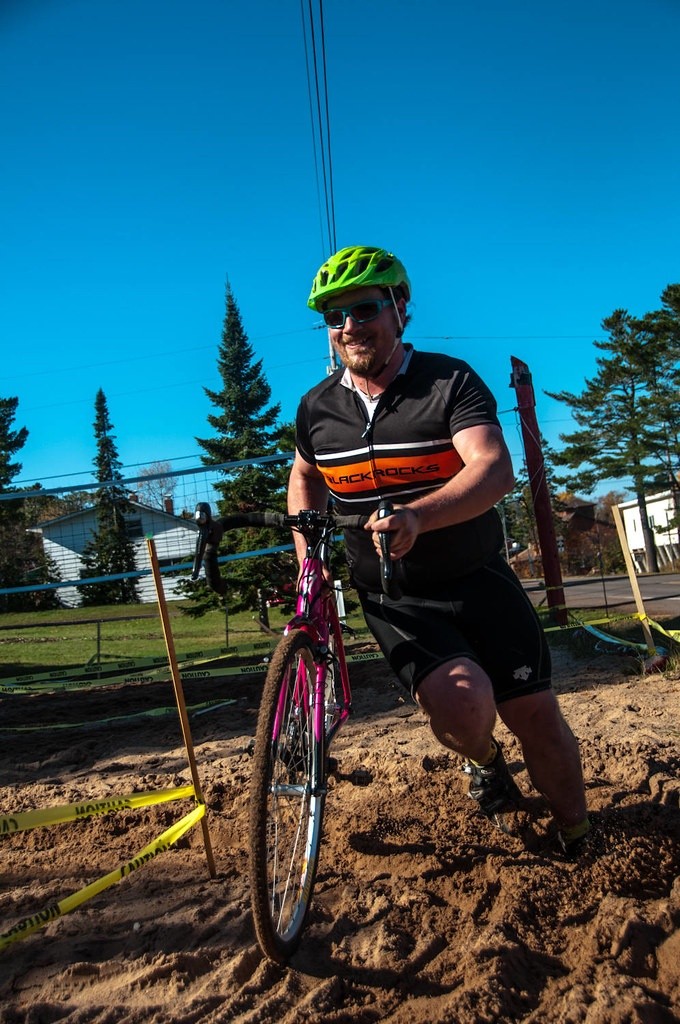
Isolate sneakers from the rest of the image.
[462,734,529,833]
[549,825,597,865]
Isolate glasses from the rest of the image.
[323,298,398,329]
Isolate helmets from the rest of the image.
[307,246,411,316]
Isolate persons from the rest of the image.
[286,246,592,855]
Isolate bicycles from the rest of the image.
[186,501,399,962]
